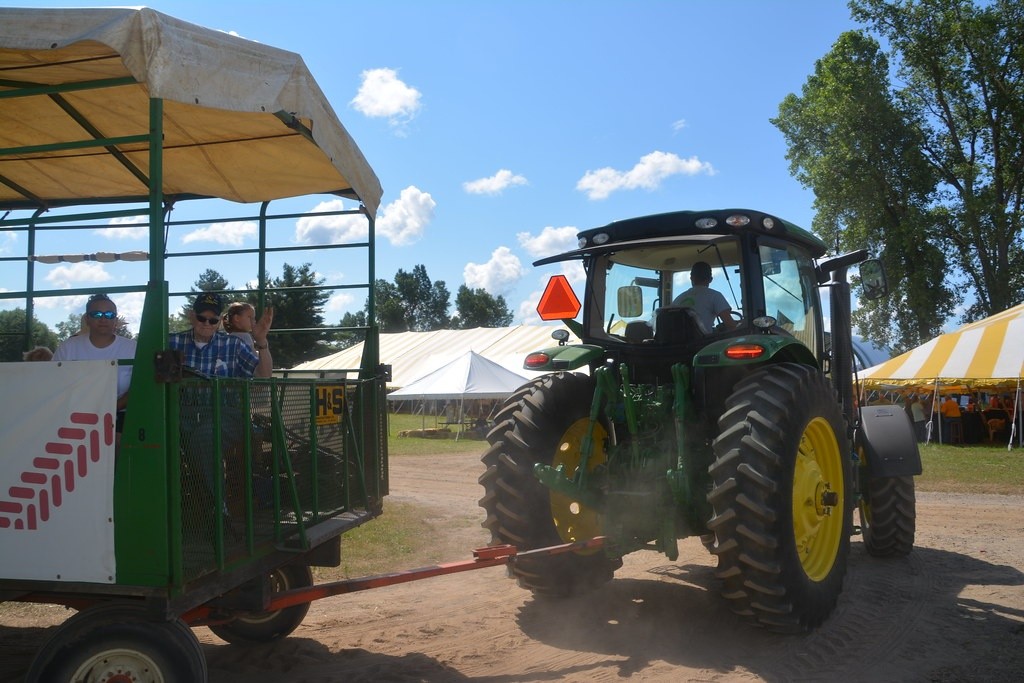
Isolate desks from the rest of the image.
[960,412,1022,444]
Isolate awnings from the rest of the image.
[289,319,628,439]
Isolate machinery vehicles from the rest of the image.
[0,7,924,683]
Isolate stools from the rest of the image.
[951,422,964,444]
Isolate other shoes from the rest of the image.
[210,499,231,534]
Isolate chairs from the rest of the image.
[652,307,709,341]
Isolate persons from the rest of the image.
[668,260,737,335]
[23,293,137,450]
[168,291,276,525]
[869,389,1024,447]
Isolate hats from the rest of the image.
[192,293,222,316]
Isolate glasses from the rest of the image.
[86,310,116,319]
[195,314,220,325]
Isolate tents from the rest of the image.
[852,303,1024,447]
